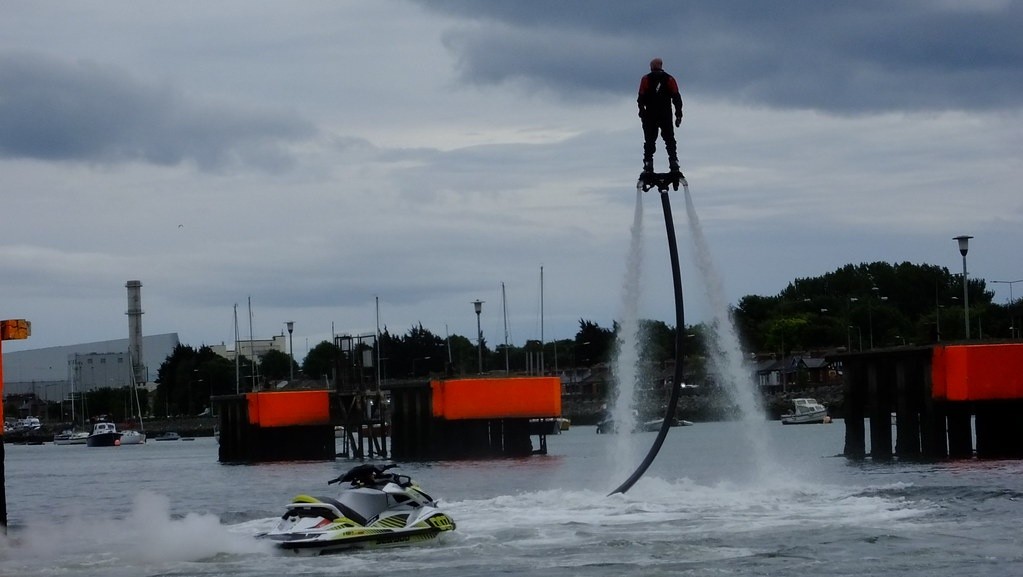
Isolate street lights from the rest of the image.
[673,333,696,380]
[990,279,1023,342]
[953,235,975,341]
[282,321,296,385]
[568,340,591,400]
[935,271,970,342]
[781,298,812,396]
[847,296,859,354]
[412,356,432,380]
[869,286,880,350]
[469,300,487,378]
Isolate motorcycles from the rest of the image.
[594,412,639,435]
[253,462,458,559]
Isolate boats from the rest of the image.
[643,414,693,433]
[780,398,833,426]
[154,431,181,441]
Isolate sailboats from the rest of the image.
[213,295,264,445]
[526,340,572,431]
[5,345,146,447]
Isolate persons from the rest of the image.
[637,57,683,173]
